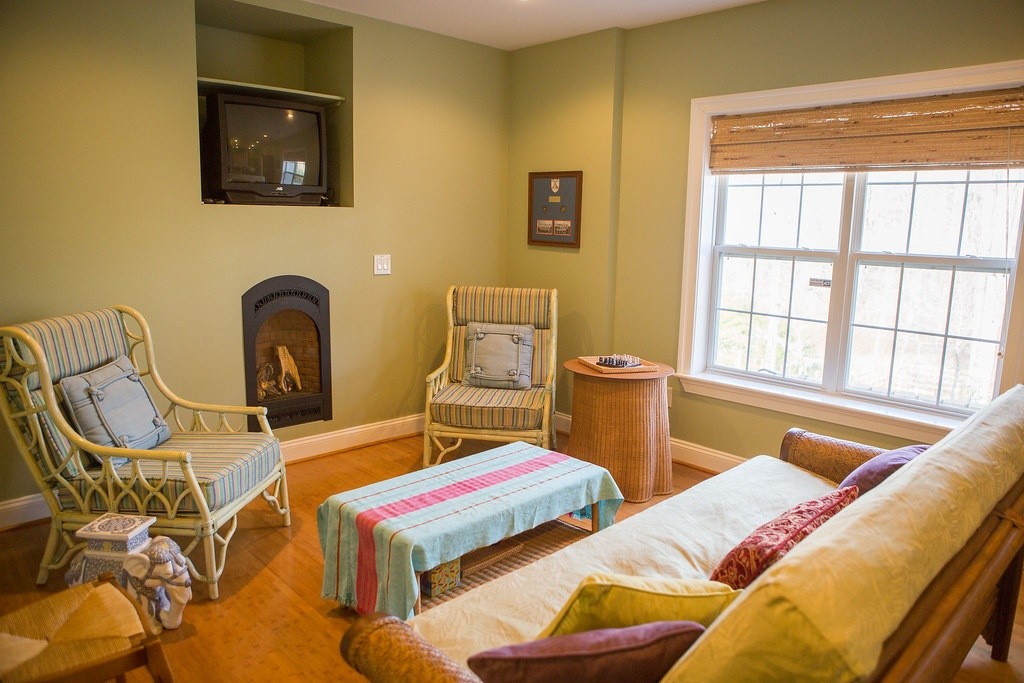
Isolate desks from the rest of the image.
[563,359,675,503]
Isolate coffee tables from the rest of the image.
[317,441,625,622]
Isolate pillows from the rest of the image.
[59,355,171,469]
[467,621,706,683]
[535,574,743,629]
[461,322,535,391]
[710,485,860,591]
[837,445,931,497]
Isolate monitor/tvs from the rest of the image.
[198,92,329,207]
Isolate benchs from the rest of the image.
[339,382,1023,683]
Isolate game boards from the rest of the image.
[578,353,659,373]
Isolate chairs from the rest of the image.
[0,306,291,600]
[422,286,558,467]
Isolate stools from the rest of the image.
[1,571,175,683]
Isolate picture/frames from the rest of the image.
[528,171,583,247]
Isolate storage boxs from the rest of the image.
[420,557,461,597]
